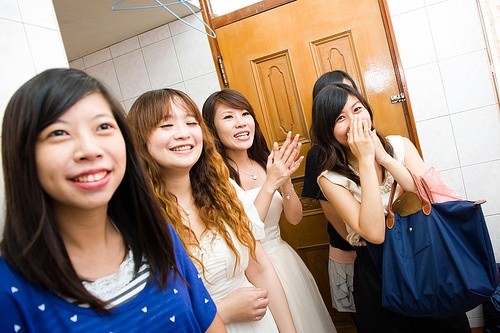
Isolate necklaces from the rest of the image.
[236,158,258,180]
[177,192,193,215]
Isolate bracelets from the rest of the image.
[280,184,296,202]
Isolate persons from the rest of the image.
[0,68,227,333]
[316,81,468,333]
[125,88,297,333]
[301,70,359,333]
[202,89,339,333]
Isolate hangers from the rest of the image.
[154,0,217,39]
[110,0,188,10]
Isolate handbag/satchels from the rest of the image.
[383,167,499,317]
